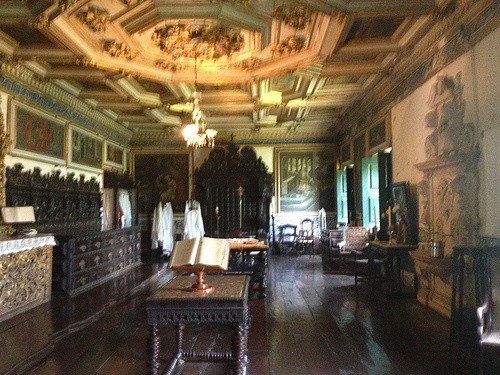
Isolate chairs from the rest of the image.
[473,245,500,375]
[278,217,315,255]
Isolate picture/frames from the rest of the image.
[104,139,127,170]
[66,121,105,174]
[363,114,391,157]
[274,146,338,216]
[129,149,193,218]
[339,139,353,164]
[6,94,68,166]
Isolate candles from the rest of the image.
[389,206,391,227]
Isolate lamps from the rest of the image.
[181,48,217,148]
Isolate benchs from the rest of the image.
[320,226,370,271]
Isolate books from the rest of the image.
[169,237,230,270]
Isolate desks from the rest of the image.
[224,233,270,299]
[370,241,419,298]
[146,273,253,375]
